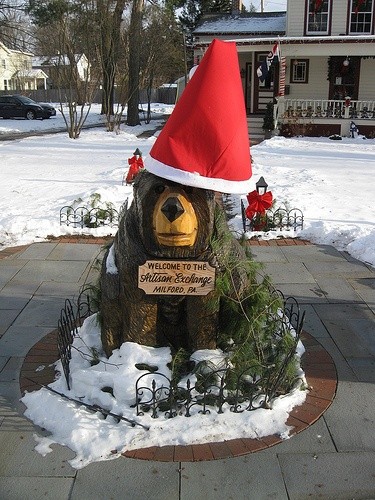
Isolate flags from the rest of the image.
[257,34,286,103]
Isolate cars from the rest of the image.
[21,95,56,119]
[0,95,44,120]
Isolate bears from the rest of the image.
[98,171,250,374]
